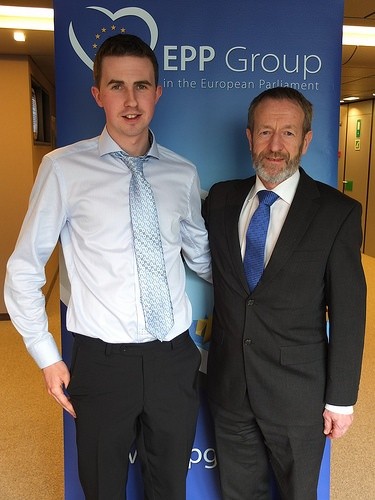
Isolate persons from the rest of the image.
[4,33,213,500]
[201,87,367,500]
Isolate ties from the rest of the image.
[243,190,279,293]
[110,150,175,342]
